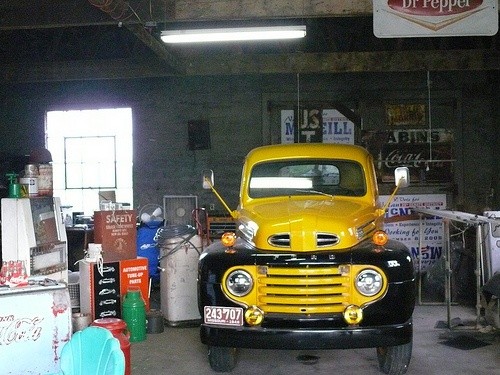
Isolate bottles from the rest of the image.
[19,162,40,196]
[0,259,27,282]
[37,161,54,196]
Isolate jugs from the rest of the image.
[145,308,165,335]
[89,318,131,375]
[121,289,147,342]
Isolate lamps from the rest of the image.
[160,0,307,44]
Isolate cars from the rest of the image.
[196,141,418,374]
